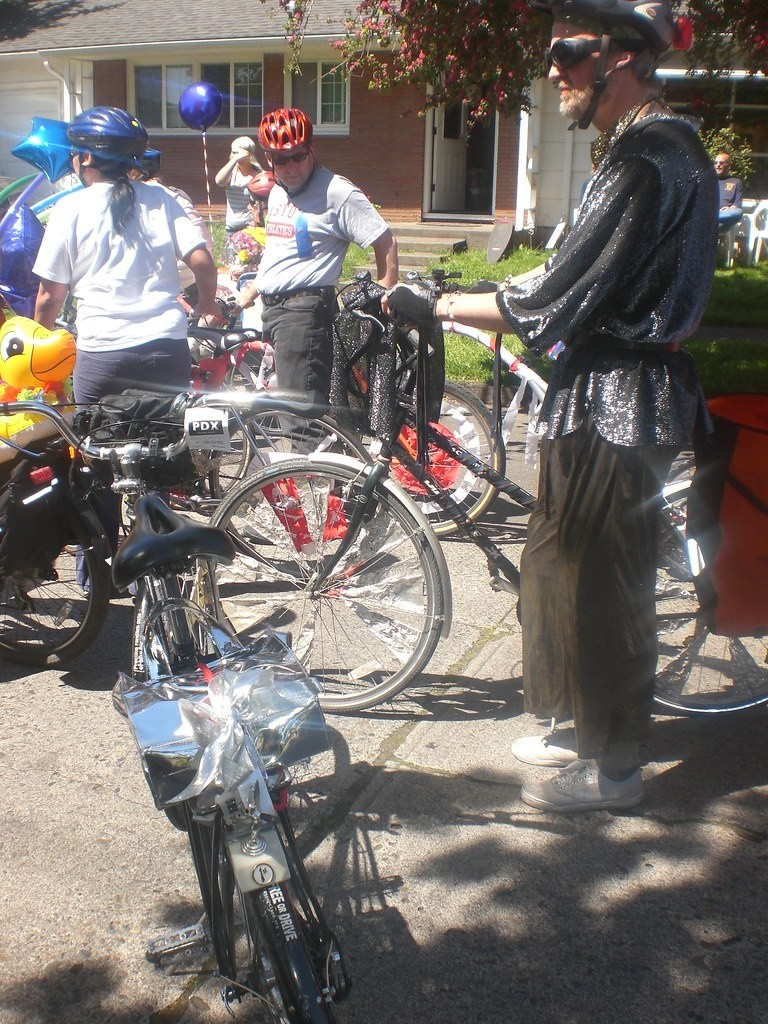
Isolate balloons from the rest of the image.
[12,116,79,182]
[0,201,45,320]
[179,81,221,133]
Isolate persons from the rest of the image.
[30,107,227,599]
[380,0,721,813]
[215,136,260,268]
[127,145,217,292]
[216,172,277,297]
[229,108,398,491]
[713,152,743,235]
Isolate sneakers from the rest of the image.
[521,758,645,814]
[511,717,581,766]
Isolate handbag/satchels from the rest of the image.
[111,596,331,811]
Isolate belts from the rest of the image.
[261,285,335,305]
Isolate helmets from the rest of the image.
[246,171,278,201]
[143,146,161,160]
[530,0,676,59]
[258,107,313,153]
[67,105,149,144]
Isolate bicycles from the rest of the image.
[0,397,354,1024]
[0,266,768,730]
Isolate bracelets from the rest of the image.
[447,289,463,321]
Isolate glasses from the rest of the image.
[544,37,593,72]
[273,150,310,165]
[249,199,255,206]
[70,143,78,156]
